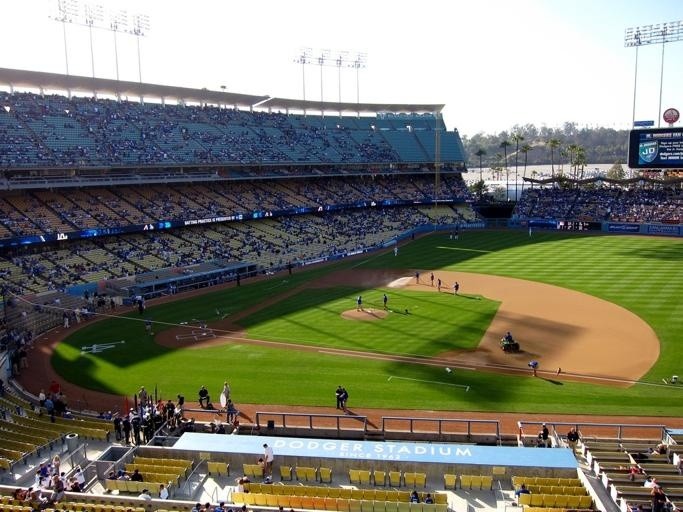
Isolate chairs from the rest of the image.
[356,112,481,255]
[193,105,355,289]
[0,346,35,511]
[0,88,193,342]
[36,395,683,511]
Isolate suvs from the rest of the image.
[499,337,519,352]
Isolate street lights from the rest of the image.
[46,0,151,84]
[623,17,682,132]
[291,45,369,116]
